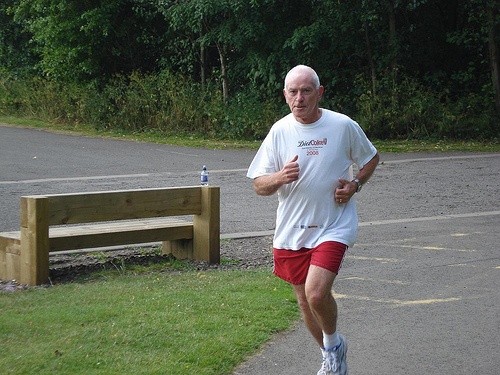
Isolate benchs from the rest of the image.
[0,185,220,286]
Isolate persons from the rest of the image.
[245,64,381,375]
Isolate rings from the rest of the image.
[339,198,343,203]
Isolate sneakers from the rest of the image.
[315,335,349,375]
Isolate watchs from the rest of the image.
[350,178,362,192]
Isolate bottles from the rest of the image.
[201,165,208,186]
